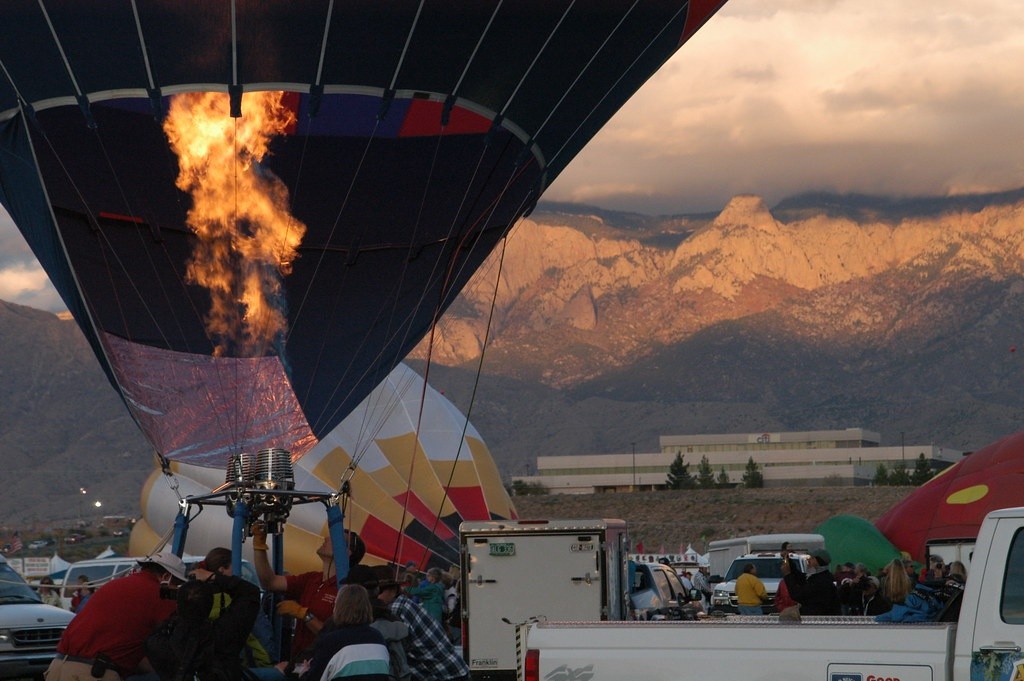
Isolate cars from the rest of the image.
[0,552,78,681]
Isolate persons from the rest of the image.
[34,531,471,681]
[678,541,968,623]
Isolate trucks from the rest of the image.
[708,532,825,580]
[457,517,628,674]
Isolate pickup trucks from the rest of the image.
[522,505,1024,681]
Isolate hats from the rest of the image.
[135,551,188,585]
[364,566,402,590]
[806,548,832,566]
[699,559,711,568]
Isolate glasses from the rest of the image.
[378,584,386,595]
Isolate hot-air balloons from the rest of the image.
[0,2,732,677]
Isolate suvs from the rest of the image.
[711,550,814,609]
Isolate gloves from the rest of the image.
[250,522,270,550]
[276,599,309,620]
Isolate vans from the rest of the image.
[57,555,260,613]
[627,560,703,622]
